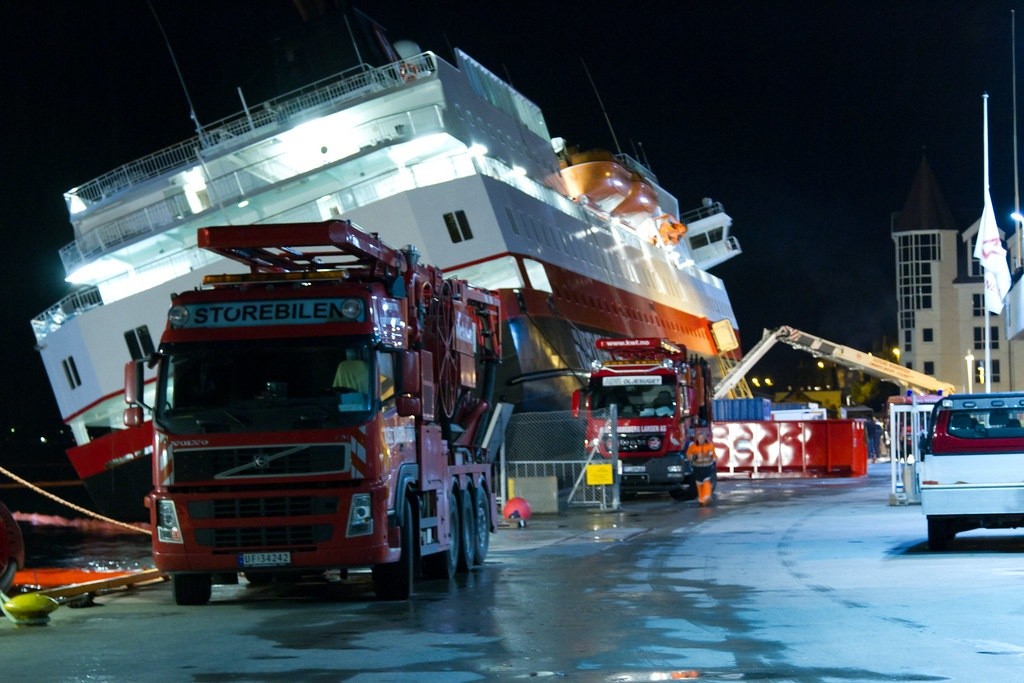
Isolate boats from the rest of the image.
[34,31,740,517]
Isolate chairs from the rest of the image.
[950,411,1024,439]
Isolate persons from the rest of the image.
[883,417,891,457]
[684,433,718,507]
[863,414,879,457]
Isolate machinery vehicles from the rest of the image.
[712,325,955,462]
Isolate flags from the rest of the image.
[971,122,1014,317]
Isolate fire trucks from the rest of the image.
[124,216,501,607]
[570,339,716,505]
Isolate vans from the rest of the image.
[919,394,1024,544]
